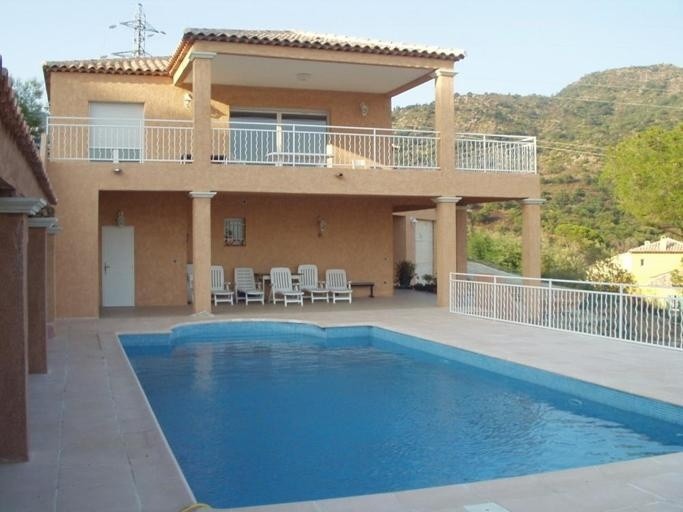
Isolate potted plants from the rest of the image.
[395,260,415,288]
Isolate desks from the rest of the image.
[254,272,303,305]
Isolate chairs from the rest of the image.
[211,265,234,307]
[326,269,353,303]
[234,268,265,306]
[270,267,304,307]
[298,264,329,304]
